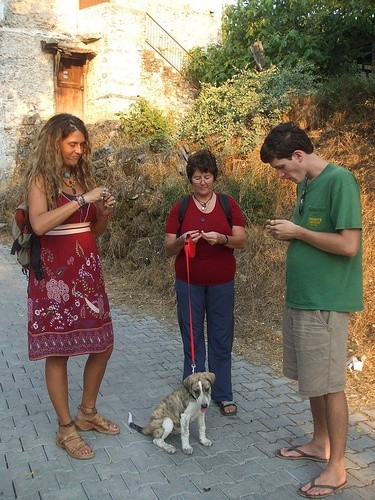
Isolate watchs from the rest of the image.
[222,235,228,245]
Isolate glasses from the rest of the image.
[299,186,306,216]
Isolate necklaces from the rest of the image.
[194,193,213,210]
[62,172,77,194]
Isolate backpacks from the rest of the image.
[11,171,58,270]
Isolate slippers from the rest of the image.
[215,401,237,416]
[275,445,329,462]
[296,475,348,499]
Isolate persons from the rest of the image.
[26,113,121,459]
[261,122,363,498]
[165,150,248,417]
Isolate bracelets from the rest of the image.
[74,195,85,207]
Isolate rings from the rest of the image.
[102,197,104,200]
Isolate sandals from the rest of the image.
[75,406,120,434]
[56,422,94,459]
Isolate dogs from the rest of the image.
[127,372,216,455]
